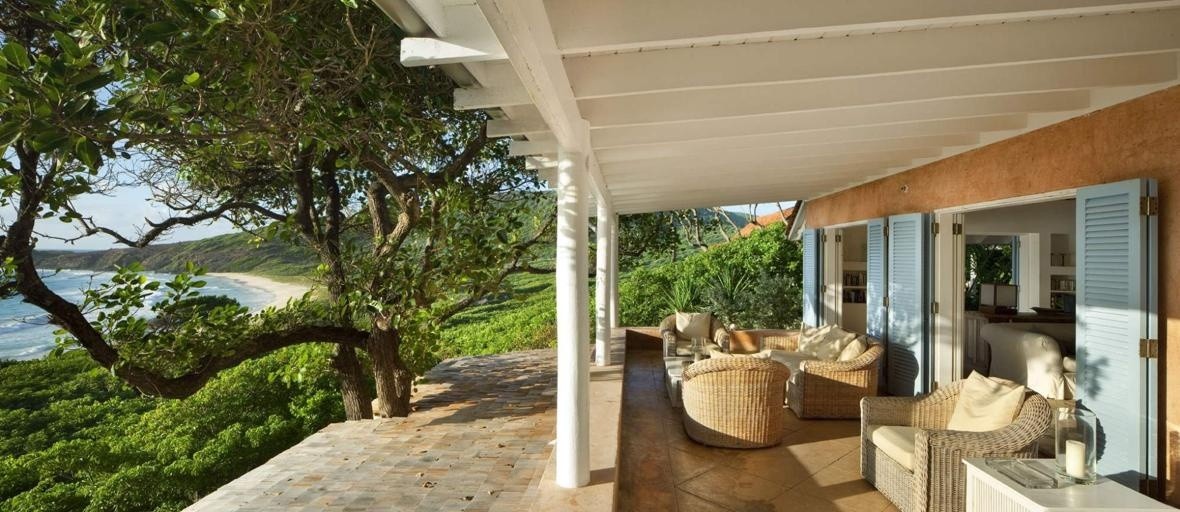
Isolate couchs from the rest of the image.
[759,326,886,421]
[980,322,1076,401]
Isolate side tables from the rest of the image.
[960,458,1180,512]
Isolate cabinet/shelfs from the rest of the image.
[1050,266,1076,311]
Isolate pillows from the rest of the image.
[947,369,1025,432]
[1062,356,1075,372]
[794,322,868,362]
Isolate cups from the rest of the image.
[690,336,707,363]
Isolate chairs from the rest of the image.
[859,376,1052,512]
[659,313,791,450]
